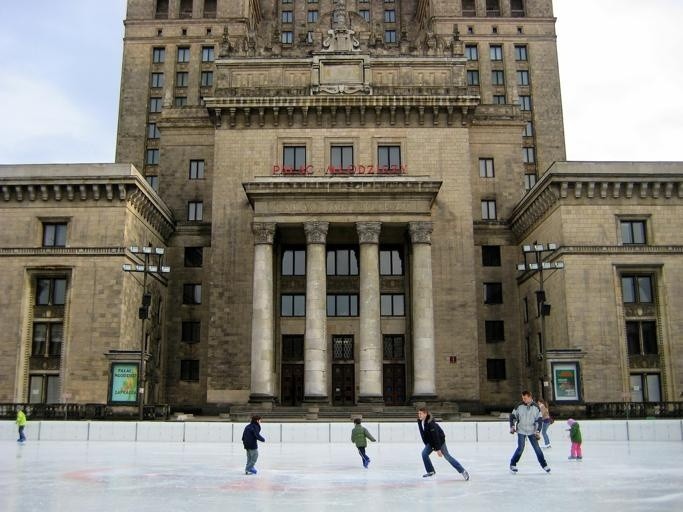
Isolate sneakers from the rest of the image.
[18,437,25,442]
[461,470,468,478]
[245,467,256,473]
[422,470,434,477]
[364,460,369,467]
[510,444,582,473]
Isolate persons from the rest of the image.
[537,399,551,448]
[510,391,550,472]
[417,408,469,480]
[15,405,26,442]
[566,418,582,459]
[351,418,376,468]
[242,415,265,474]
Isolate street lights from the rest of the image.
[516,240,565,411]
[121,243,172,420]
